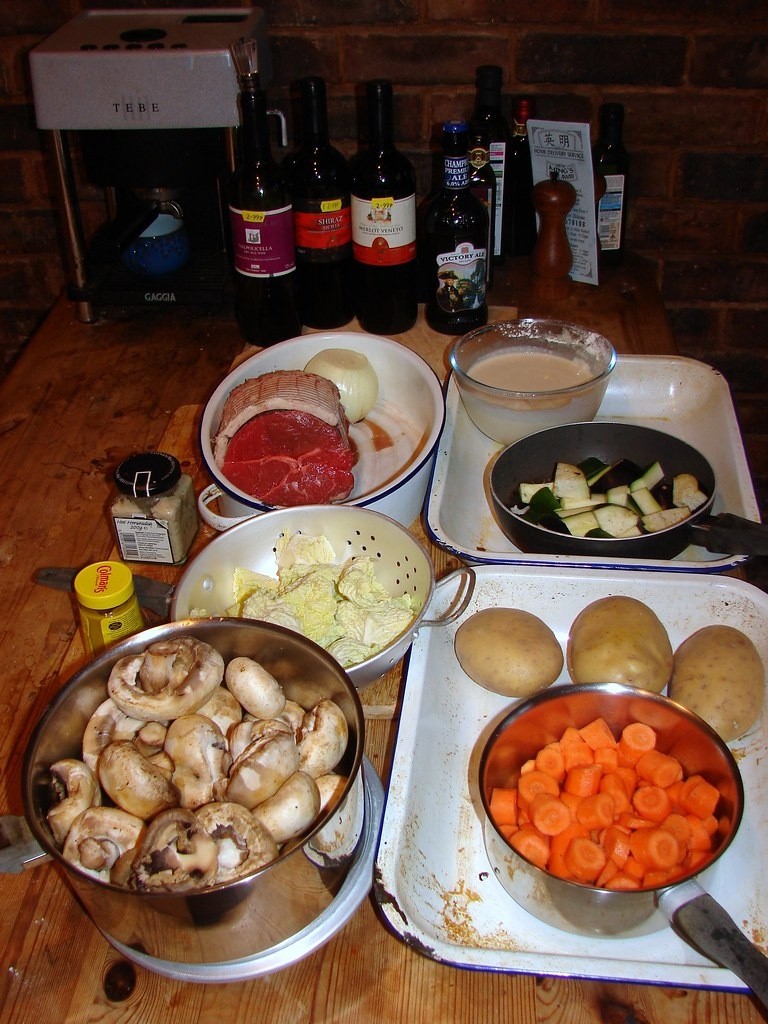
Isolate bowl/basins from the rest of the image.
[448,318,617,445]
[197,331,446,529]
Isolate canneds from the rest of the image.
[75,562,144,656]
[108,451,200,565]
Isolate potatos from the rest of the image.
[456,595,764,742]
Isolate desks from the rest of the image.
[0,256,768,1024]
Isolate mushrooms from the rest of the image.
[49,636,348,892]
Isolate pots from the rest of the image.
[33,505,476,692]
[1,617,364,902]
[478,681,767,1017]
[488,422,768,559]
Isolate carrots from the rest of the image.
[487,716,720,889]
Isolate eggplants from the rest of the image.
[515,456,707,539]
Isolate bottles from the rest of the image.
[292,77,354,329]
[416,124,443,303]
[465,129,496,290]
[350,78,417,334]
[508,99,536,257]
[529,171,577,279]
[111,451,201,565]
[588,104,629,268]
[123,186,192,281]
[426,122,489,335]
[227,37,296,293]
[72,561,146,659]
[469,66,510,264]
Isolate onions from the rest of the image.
[304,349,378,423]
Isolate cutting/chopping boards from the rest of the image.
[54,403,433,721]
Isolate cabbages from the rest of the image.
[226,529,413,667]
